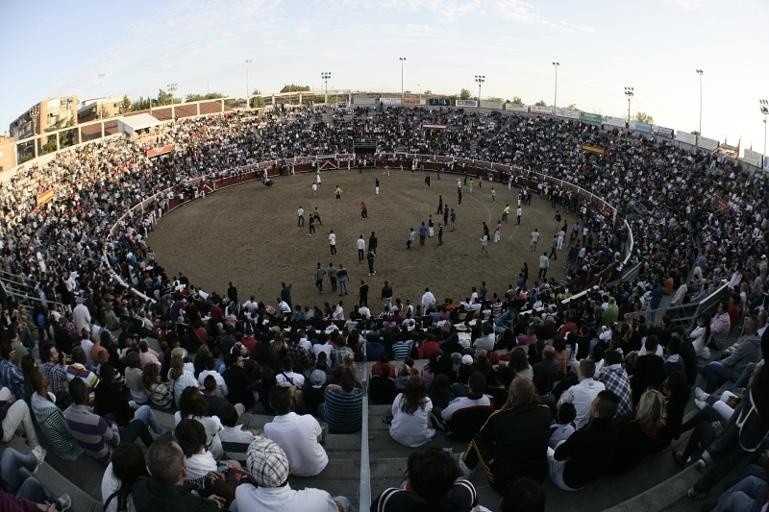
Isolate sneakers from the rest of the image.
[31,445,46,473]
[694,387,710,400]
[693,399,707,409]
[51,492,72,511]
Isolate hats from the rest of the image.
[462,353,474,365]
[170,347,189,361]
[310,368,327,389]
[247,436,289,488]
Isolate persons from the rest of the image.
[1,102,769,511]
